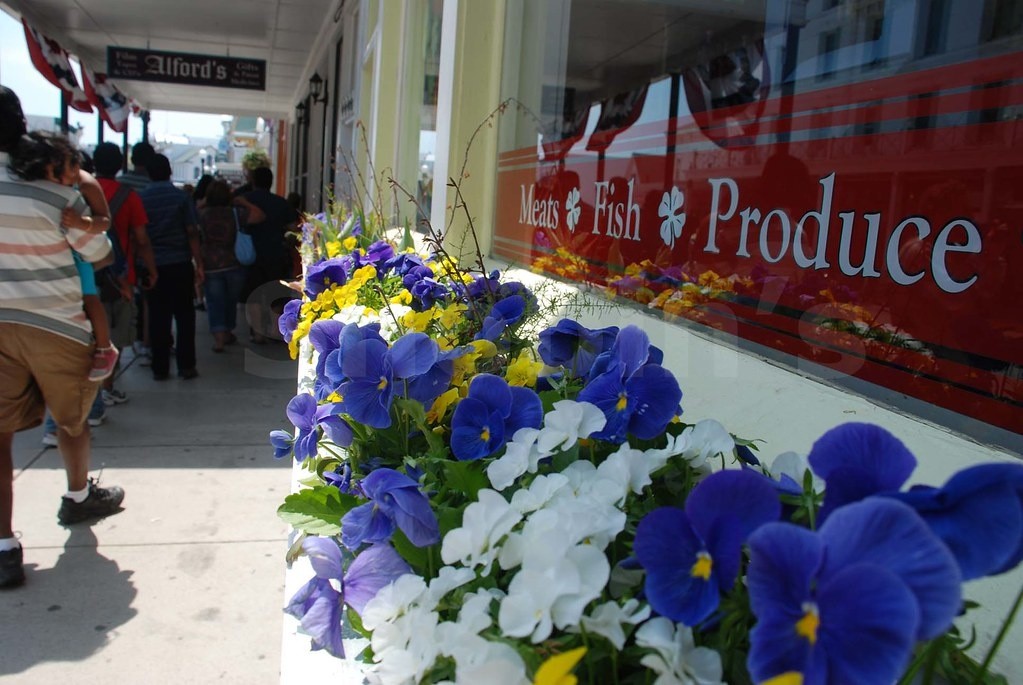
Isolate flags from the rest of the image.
[19,17,94,113]
[78,55,148,133]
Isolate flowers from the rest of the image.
[279,96,1023,685]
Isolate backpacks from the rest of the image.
[94,184,133,304]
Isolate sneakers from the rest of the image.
[58,484,124,523]
[43,433,57,446]
[103,389,130,405]
[1,542,24,585]
[88,411,106,426]
[88,342,119,382]
[138,344,154,366]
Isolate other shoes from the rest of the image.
[152,371,198,380]
[194,299,204,309]
[250,335,264,343]
[212,335,238,352]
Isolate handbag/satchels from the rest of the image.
[233,208,257,267]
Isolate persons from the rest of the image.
[117,138,162,367]
[231,163,299,345]
[84,139,157,408]
[176,164,304,310]
[41,385,110,450]
[74,147,96,176]
[2,126,120,383]
[193,173,265,355]
[0,82,125,588]
[531,136,1022,439]
[135,152,206,386]
